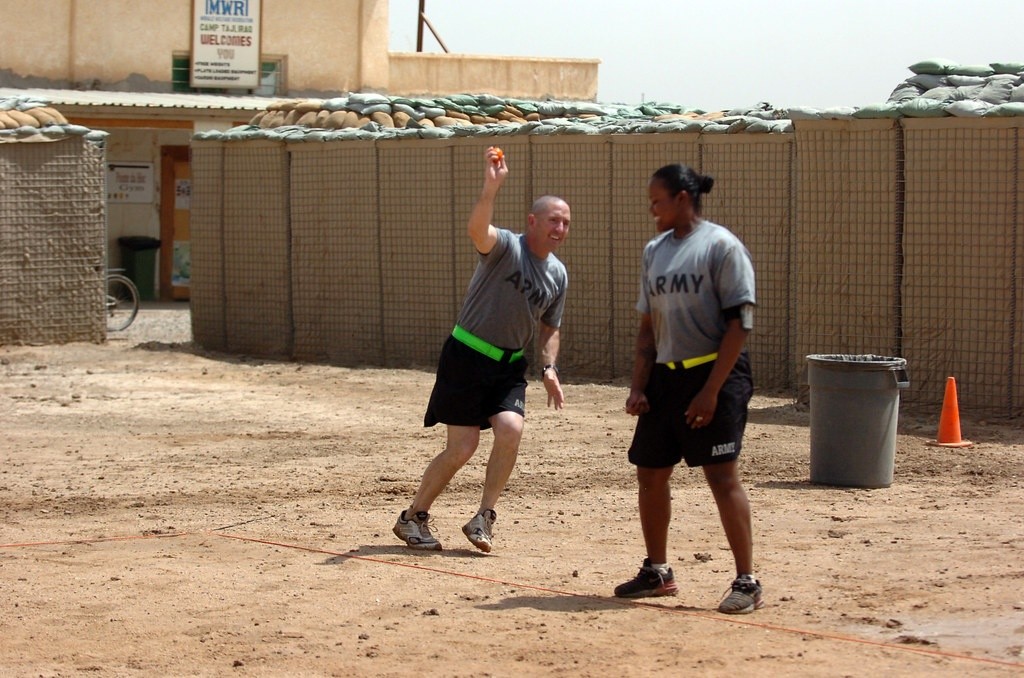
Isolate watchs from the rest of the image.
[542,364,559,377]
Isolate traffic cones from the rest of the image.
[927,377,973,448]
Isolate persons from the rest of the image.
[392,146,570,553]
[614,164,766,615]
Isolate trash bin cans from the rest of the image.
[117,235,161,301]
[805,354,910,489]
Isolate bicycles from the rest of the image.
[105,267,140,333]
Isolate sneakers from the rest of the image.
[393,510,443,550]
[614,557,678,598]
[463,509,497,552]
[719,579,764,613]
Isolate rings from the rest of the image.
[696,416,703,421]
